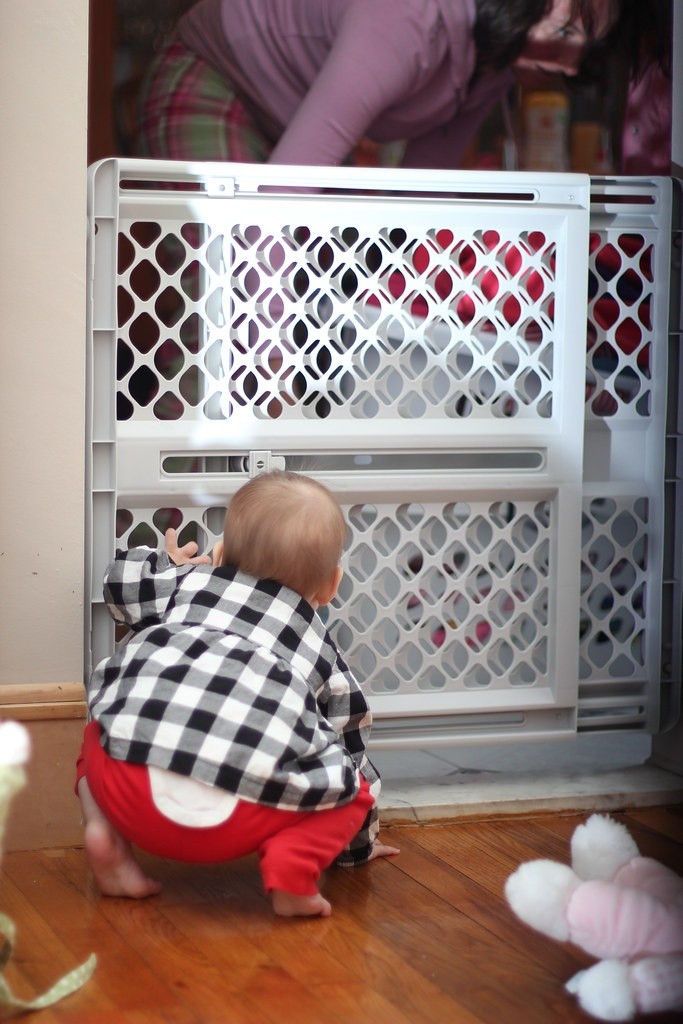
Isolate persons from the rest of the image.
[115,1,648,565]
[65,472,402,919]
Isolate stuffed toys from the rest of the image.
[499,814,682,1023]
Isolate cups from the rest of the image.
[524,90,568,172]
